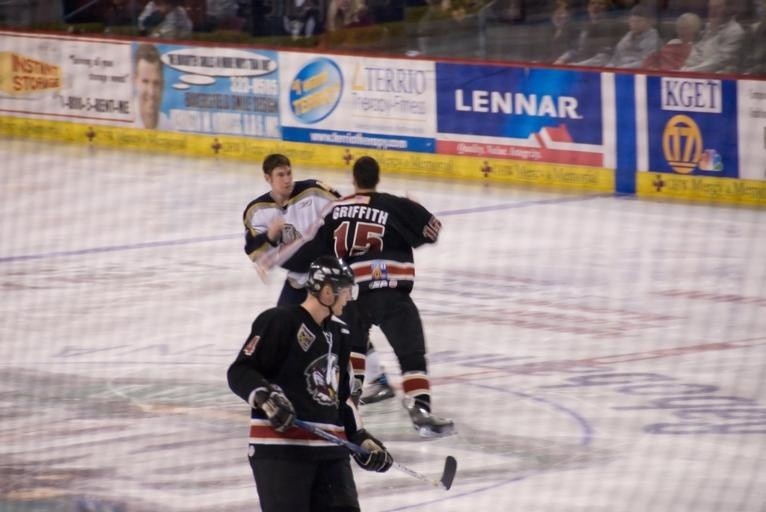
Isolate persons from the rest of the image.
[223,252,393,512]
[131,42,178,134]
[1,2,765,81]
[251,156,454,436]
[240,152,393,401]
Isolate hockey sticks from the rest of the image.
[292,420,457,490]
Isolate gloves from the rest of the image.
[349,428,394,474]
[253,383,295,433]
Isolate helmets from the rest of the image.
[306,255,355,295]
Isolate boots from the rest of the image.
[401,395,454,435]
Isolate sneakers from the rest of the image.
[356,374,396,404]
